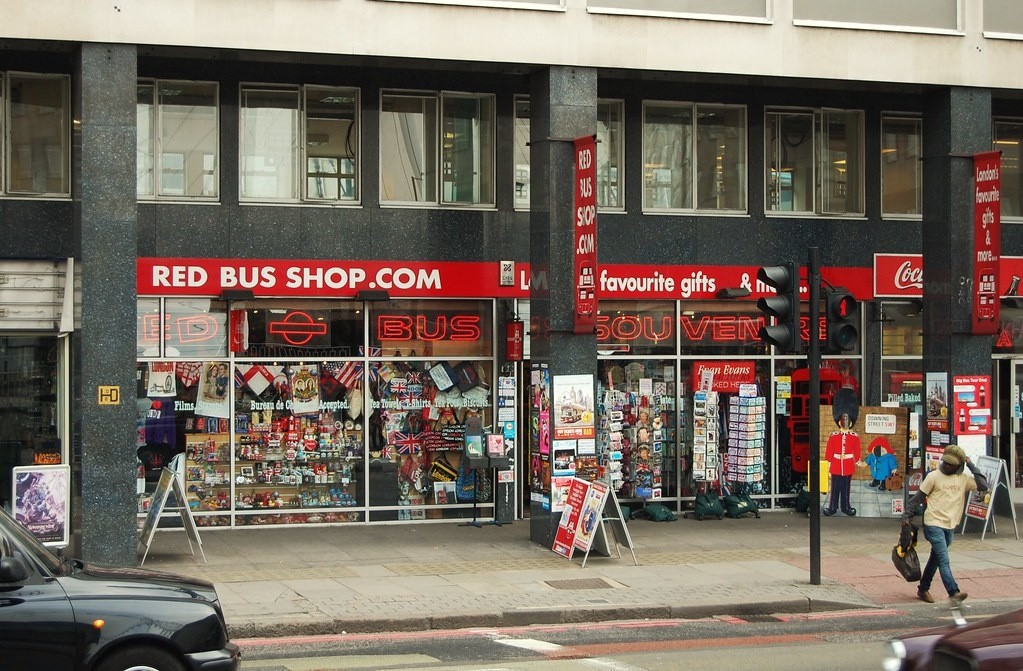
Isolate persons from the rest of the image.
[901,444,989,610]
[215,363,228,398]
[295,380,304,393]
[637,427,650,445]
[205,365,218,400]
[637,444,650,463]
[305,378,314,393]
[636,410,650,426]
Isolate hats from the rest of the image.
[940,444,967,465]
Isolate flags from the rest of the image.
[235,372,245,390]
[354,362,379,383]
[325,361,361,391]
[380,370,424,463]
[235,364,284,396]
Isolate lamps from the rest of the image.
[819,286,849,299]
[715,287,750,299]
[353,290,393,302]
[210,288,255,302]
[1001,297,1023,310]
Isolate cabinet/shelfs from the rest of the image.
[185,432,362,524]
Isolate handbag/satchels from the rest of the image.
[457,455,479,502]
[402,456,422,485]
[452,361,479,392]
[377,364,423,406]
[475,470,492,503]
[428,453,457,482]
[395,426,422,453]
[442,411,467,441]
[424,409,464,452]
[891,518,921,582]
[429,361,459,392]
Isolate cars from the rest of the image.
[881,607,1023,671]
[0,502,240,671]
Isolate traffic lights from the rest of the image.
[755,260,802,354]
[825,289,859,352]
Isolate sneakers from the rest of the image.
[948,592,968,601]
[917,585,935,603]
